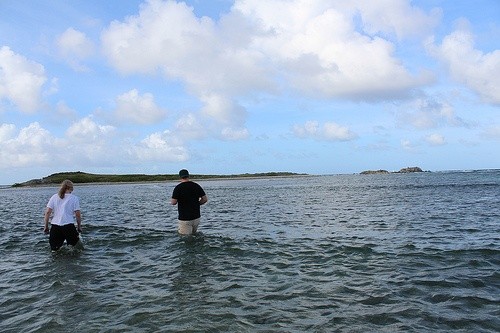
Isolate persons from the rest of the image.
[43,178,83,256]
[170,169,207,236]
